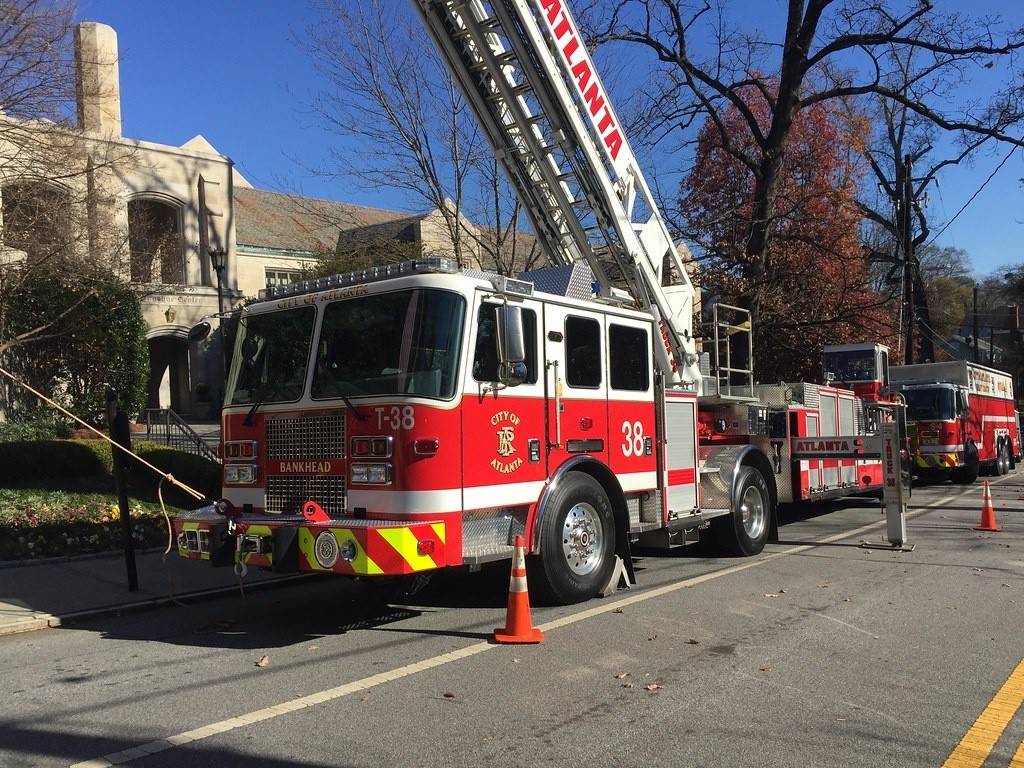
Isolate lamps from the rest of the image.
[165,306,177,321]
[823,372,834,386]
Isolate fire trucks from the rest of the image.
[820,341,1019,486]
[171,0,920,608]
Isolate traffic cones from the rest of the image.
[973,480,1004,531]
[494,533,544,644]
